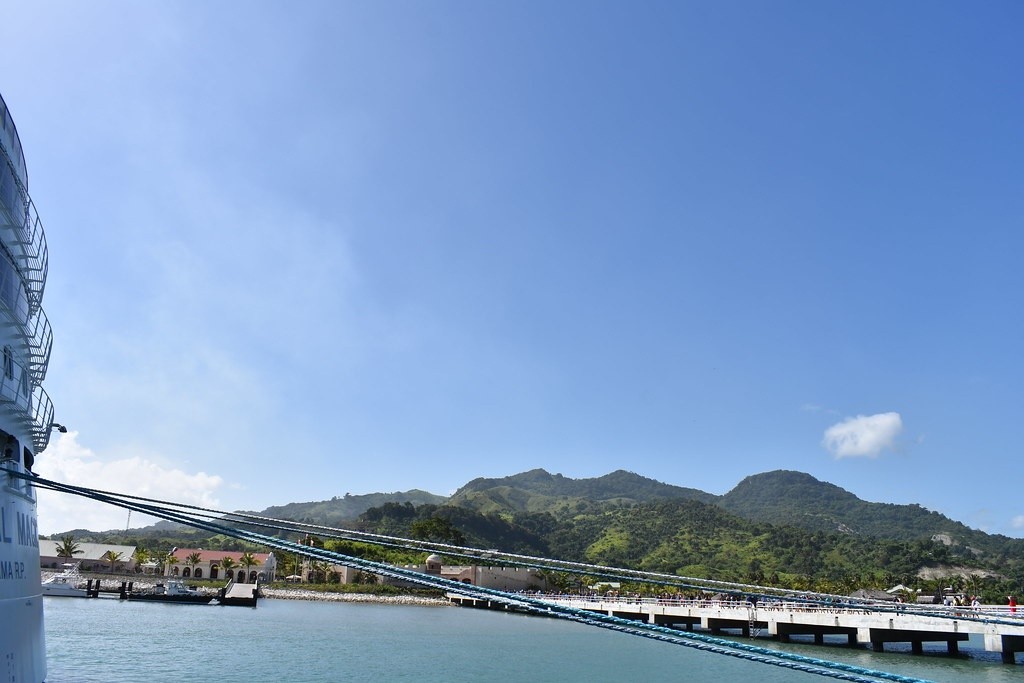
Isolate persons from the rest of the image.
[1008,596,1016,612]
[508,589,906,615]
[971,597,980,618]
[943,595,969,617]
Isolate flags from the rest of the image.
[298,533,314,546]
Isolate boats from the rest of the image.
[125,570,220,603]
[41,558,94,598]
[0,87,66,682]
